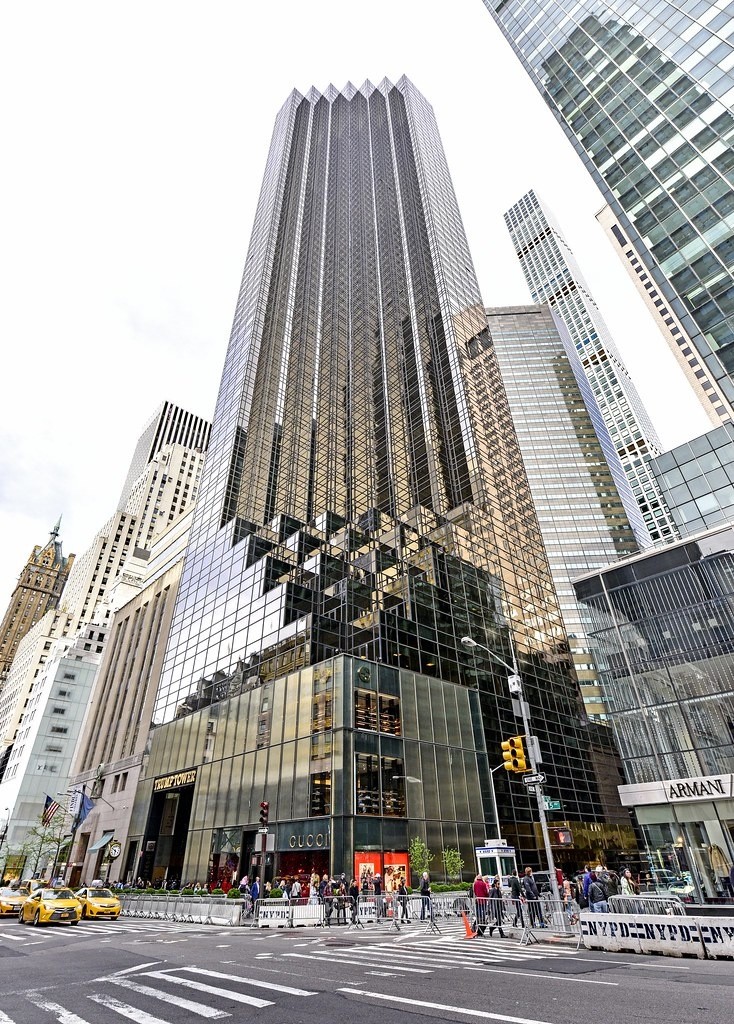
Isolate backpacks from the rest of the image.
[239,884,246,893]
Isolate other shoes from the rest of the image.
[350,918,355,924]
[611,932,615,936]
[602,932,607,936]
[489,927,493,937]
[407,920,411,924]
[501,934,508,938]
[401,920,405,923]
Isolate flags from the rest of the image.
[42,795,60,826]
[71,793,95,833]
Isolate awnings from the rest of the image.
[87,832,114,853]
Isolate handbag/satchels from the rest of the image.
[297,892,302,897]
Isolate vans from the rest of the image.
[19,879,50,894]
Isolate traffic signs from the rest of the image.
[522,772,561,811]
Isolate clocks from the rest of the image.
[104,840,121,861]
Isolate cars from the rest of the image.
[74,887,122,921]
[18,886,82,925]
[0,887,31,918]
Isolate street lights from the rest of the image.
[61,789,84,887]
[0,808,11,849]
[50,792,71,881]
[461,637,574,937]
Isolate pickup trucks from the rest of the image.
[635,869,678,892]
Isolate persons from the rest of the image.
[80,877,176,892]
[182,876,260,915]
[708,843,734,897]
[558,865,643,936]
[471,867,548,938]
[397,879,411,924]
[371,873,383,924]
[264,872,368,926]
[361,865,405,895]
[420,872,438,923]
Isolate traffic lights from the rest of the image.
[554,829,574,845]
[260,801,268,823]
[508,737,526,770]
[501,740,513,770]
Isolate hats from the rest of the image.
[113,881,118,883]
[589,873,597,881]
